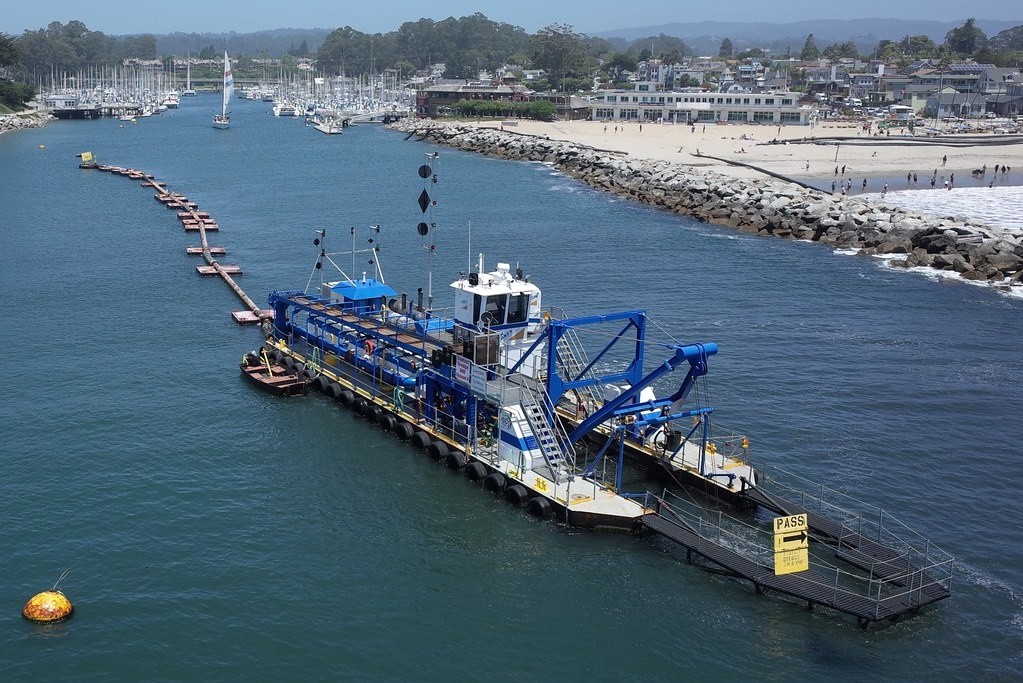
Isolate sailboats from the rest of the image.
[238,62,407,134]
[212,50,235,130]
[41,60,195,118]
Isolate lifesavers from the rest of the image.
[280,356,294,369]
[410,431,432,448]
[503,484,529,506]
[443,451,467,470]
[351,397,368,412]
[268,350,284,362]
[427,441,449,461]
[483,473,508,493]
[338,390,355,407]
[364,340,373,354]
[365,405,383,422]
[292,362,304,373]
[326,383,343,400]
[464,461,488,482]
[524,496,553,518]
[313,376,329,393]
[258,345,273,360]
[396,421,414,441]
[377,413,398,432]
[301,370,315,387]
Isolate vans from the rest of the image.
[849,98,863,107]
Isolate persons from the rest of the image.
[973,164,1010,174]
[841,185,846,195]
[842,165,846,175]
[848,178,852,190]
[913,173,918,183]
[703,125,705,132]
[615,126,617,133]
[862,178,867,190]
[832,181,835,193]
[908,173,911,182]
[640,125,642,132]
[931,169,954,189]
[881,182,888,198]
[863,125,904,137]
[691,125,695,133]
[721,133,756,153]
[806,160,809,171]
[834,166,838,176]
[604,125,607,132]
[943,155,948,166]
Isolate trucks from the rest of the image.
[815,92,827,101]
[830,95,845,105]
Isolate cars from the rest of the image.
[916,118,925,127]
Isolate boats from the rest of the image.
[265,148,750,530]
[238,345,313,396]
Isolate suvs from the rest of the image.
[981,112,997,119]
[942,116,966,124]
